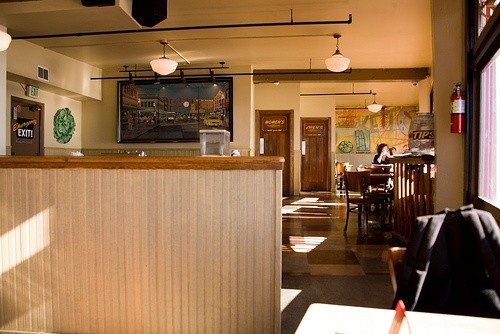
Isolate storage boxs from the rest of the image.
[199,129,231,158]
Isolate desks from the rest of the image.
[295,303,500,334]
[370,173,394,177]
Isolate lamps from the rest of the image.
[181,71,185,81]
[367,93,382,112]
[130,73,134,86]
[0,30,11,52]
[150,39,178,76]
[154,72,160,85]
[325,34,351,72]
[211,70,217,87]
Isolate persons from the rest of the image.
[373,144,396,198]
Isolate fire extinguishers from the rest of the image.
[450,84,466,134]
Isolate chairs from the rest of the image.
[343,167,393,235]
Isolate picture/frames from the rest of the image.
[117,77,233,143]
